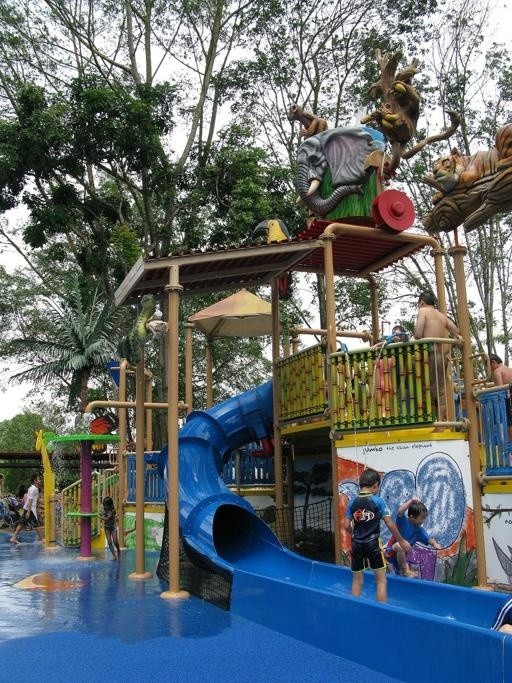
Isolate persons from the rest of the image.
[98,496,120,562]
[1,471,61,544]
[385,499,441,578]
[345,468,412,602]
[329,289,512,442]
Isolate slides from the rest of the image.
[156,378,511,683]
